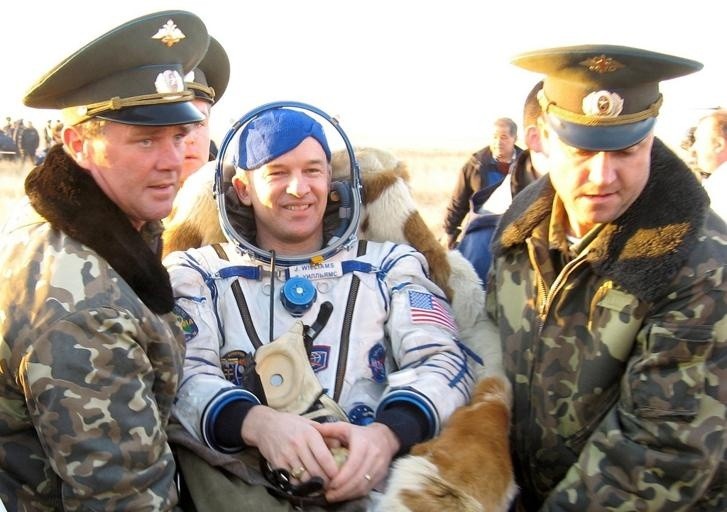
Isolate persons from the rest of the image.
[0,8,727,512]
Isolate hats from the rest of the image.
[510,43,704,151]
[22,8,210,129]
[232,109,331,170]
[184,35,230,107]
